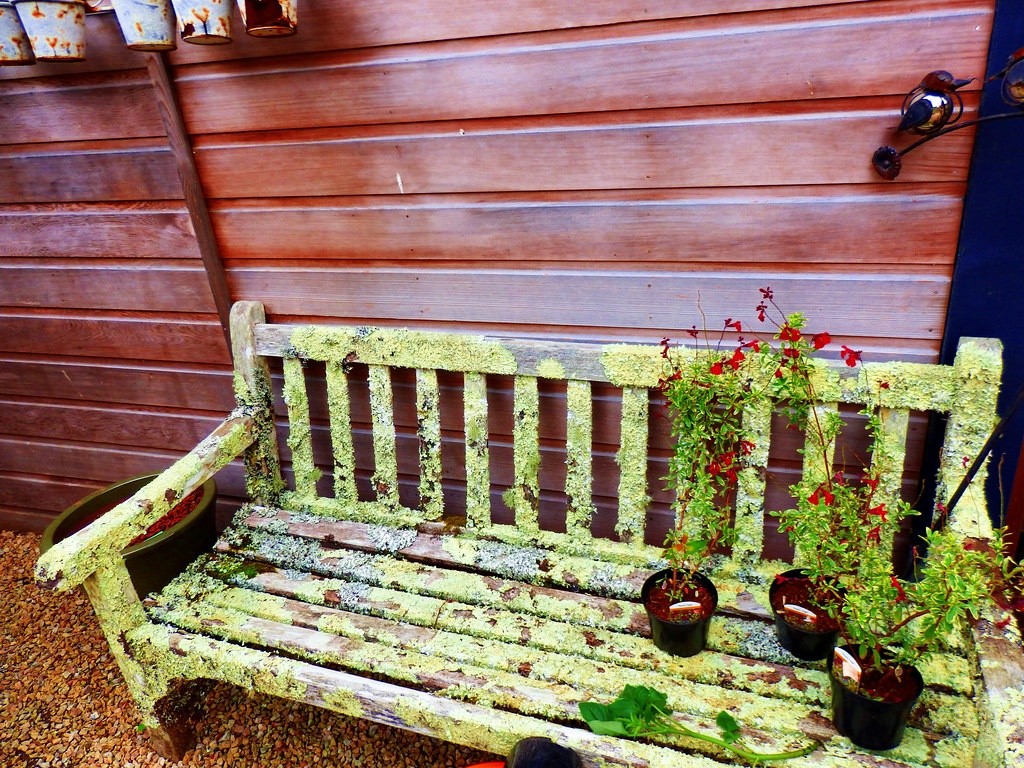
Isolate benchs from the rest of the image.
[32,301,1024,768]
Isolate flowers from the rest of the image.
[654,288,777,604]
[801,520,1024,690]
[753,284,921,615]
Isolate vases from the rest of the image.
[641,568,719,658]
[39,469,217,601]
[768,568,849,661]
[0,0,37,66]
[826,643,924,751]
[171,0,236,46]
[111,0,178,52]
[236,0,298,39]
[9,0,91,64]
